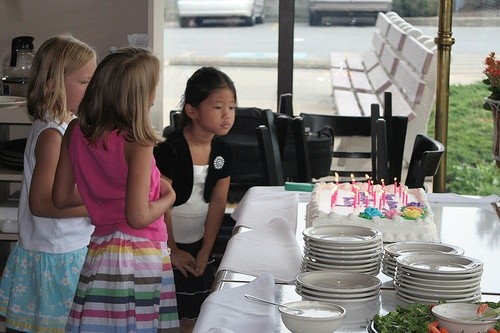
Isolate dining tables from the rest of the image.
[195,184,500,332]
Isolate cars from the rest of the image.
[175,0,265,28]
[309,0,394,26]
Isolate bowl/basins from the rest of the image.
[278,300,347,333]
[431,303,500,333]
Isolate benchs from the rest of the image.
[330,11,440,187]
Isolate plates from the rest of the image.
[366,324,374,333]
[294,224,484,304]
[370,312,389,333]
[0,96,27,109]
[0,137,27,172]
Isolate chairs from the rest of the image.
[255,90,394,184]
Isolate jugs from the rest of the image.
[2,36,38,77]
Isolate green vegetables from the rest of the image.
[371,299,500,333]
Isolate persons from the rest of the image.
[0,36,97,333]
[153,67,237,333]
[52,47,180,333]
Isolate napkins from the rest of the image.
[192,185,314,333]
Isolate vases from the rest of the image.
[484,97,500,164]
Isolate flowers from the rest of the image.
[482,52,500,100]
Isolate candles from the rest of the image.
[330,172,408,211]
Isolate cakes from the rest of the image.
[305,182,438,243]
